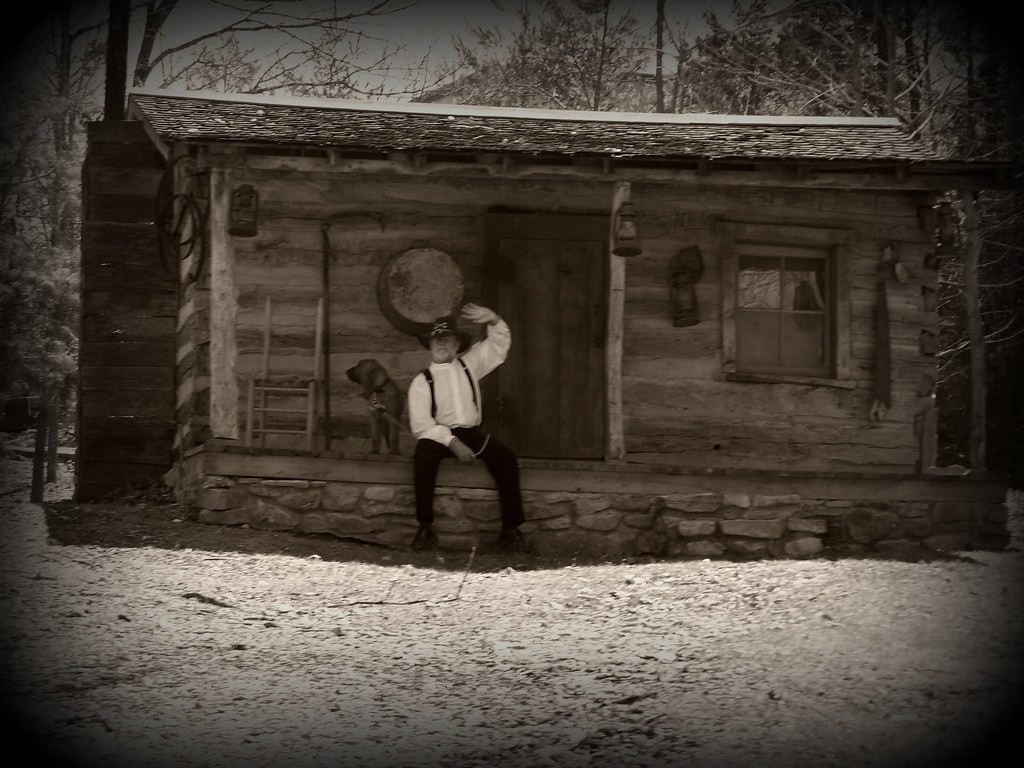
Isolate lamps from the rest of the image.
[611,201,644,258]
[226,184,260,238]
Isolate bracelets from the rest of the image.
[489,314,502,326]
[449,438,460,450]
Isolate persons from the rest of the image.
[408,303,533,549]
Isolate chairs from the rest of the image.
[244,297,325,452]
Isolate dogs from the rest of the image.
[345,358,410,456]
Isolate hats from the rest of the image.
[417,316,471,354]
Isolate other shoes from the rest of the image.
[411,525,438,553]
[499,526,531,553]
